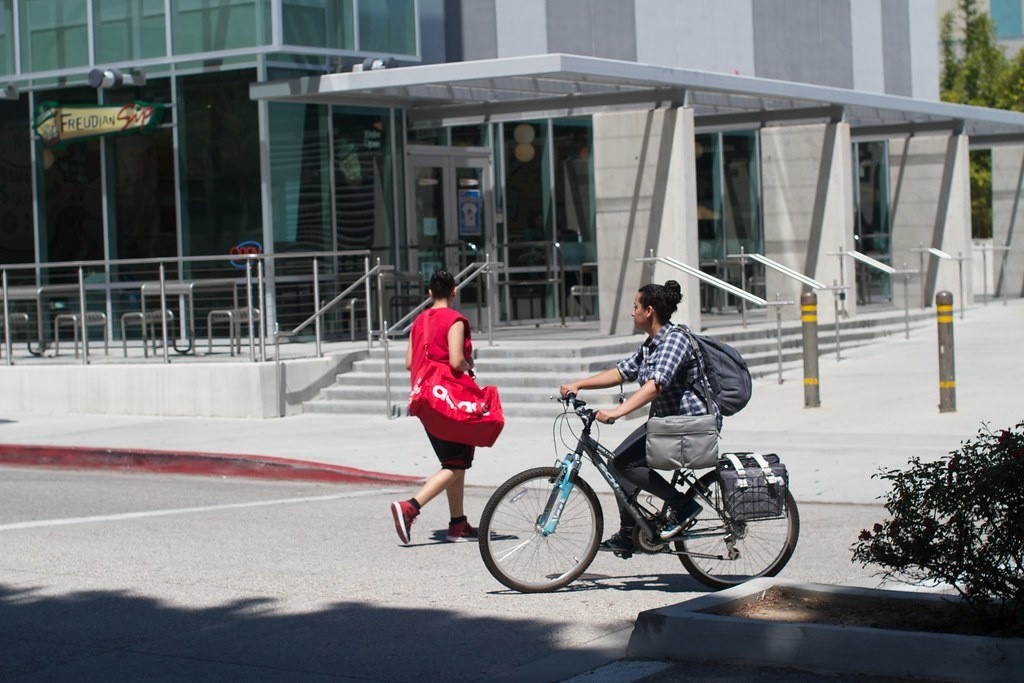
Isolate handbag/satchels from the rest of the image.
[717,452,787,521]
[645,414,720,469]
[406,361,504,448]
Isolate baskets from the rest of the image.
[715,452,789,522]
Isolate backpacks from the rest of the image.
[668,323,753,417]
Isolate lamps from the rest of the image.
[88,70,147,88]
[343,56,398,74]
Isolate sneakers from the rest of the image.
[446,517,479,541]
[660,498,702,540]
[391,500,420,544]
[596,530,642,554]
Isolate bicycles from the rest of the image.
[479,390,801,593]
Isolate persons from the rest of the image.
[560,280,723,554]
[390,271,479,543]
[558,229,591,316]
